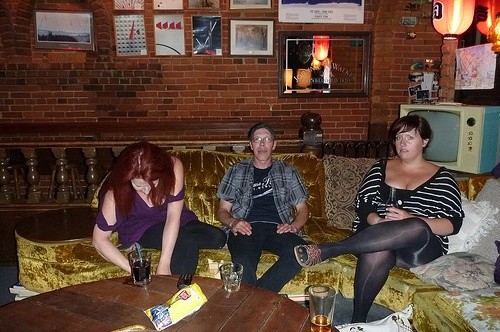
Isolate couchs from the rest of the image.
[14,149,500,332]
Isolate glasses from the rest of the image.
[251,136,274,143]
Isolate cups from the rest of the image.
[308,283,336,332]
[220,262,243,292]
[128,251,152,285]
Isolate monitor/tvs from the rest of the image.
[400,104,500,174]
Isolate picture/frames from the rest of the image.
[229,19,276,58]
[33,9,94,50]
[229,0,273,11]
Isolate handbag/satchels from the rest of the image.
[335,305,413,332]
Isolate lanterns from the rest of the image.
[313,35,330,62]
[432,0,500,39]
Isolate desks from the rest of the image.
[0,275,339,332]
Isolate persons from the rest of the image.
[215,122,309,292]
[92,144,227,277]
[294,116,465,323]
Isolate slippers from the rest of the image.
[294,244,330,268]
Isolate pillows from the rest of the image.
[446,191,499,254]
[410,251,497,292]
[470,178,500,262]
[323,154,380,230]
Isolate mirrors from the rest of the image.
[278,31,371,97]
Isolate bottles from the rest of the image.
[385,188,397,213]
